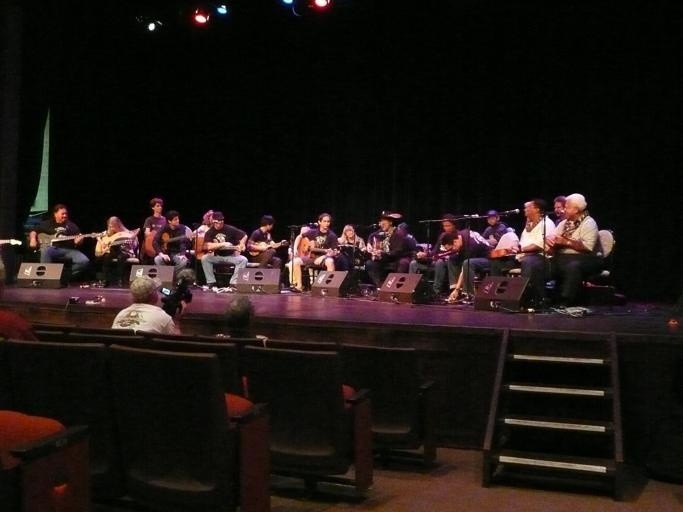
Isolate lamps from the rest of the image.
[144,0,332,32]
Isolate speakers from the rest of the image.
[16,262,68,289]
[237,267,283,293]
[474,276,533,311]
[380,273,423,303]
[311,271,352,296]
[129,264,177,290]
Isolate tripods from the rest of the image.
[178,223,212,291]
[281,224,308,293]
[448,214,497,304]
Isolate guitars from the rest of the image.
[95,228,141,257]
[249,240,288,257]
[296,238,341,259]
[36,232,98,250]
[371,236,391,261]
[145,232,194,257]
[194,238,245,259]
[414,248,455,262]
[488,248,542,260]
[545,227,572,255]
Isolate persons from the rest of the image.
[30,204,90,276]
[288,210,416,294]
[0,256,41,342]
[111,274,186,336]
[214,294,270,348]
[139,199,283,292]
[96,216,139,286]
[409,192,598,309]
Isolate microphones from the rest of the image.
[212,220,219,224]
[545,210,564,215]
[309,222,319,228]
[370,223,383,229]
[497,209,520,215]
[100,231,109,236]
[464,213,480,219]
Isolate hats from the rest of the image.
[130,275,162,300]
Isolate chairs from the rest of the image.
[107,342,272,512]
[243,345,375,505]
[574,228,618,314]
[2,409,122,511]
[3,338,109,419]
[339,343,438,471]
[152,337,248,410]
[32,325,337,355]
